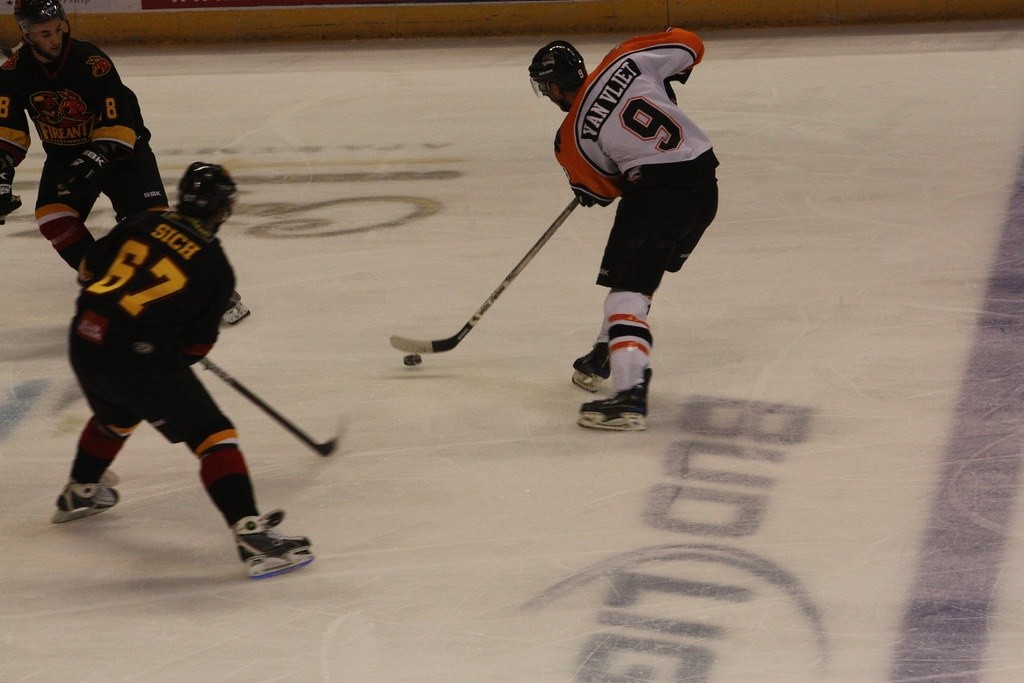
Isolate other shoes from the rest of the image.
[226,294,251,325]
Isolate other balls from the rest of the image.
[403,354,423,366]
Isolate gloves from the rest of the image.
[0,166,22,226]
[70,146,108,179]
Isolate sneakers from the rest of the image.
[578,369,652,431]
[52,468,120,525]
[232,510,314,579]
[572,343,611,394]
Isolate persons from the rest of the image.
[49,161,315,580]
[528,27,720,430]
[0,0,251,326]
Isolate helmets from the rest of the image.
[528,40,588,93]
[177,161,237,217]
[13,0,66,39]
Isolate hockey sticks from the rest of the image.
[390,196,580,354]
[201,357,353,457]
